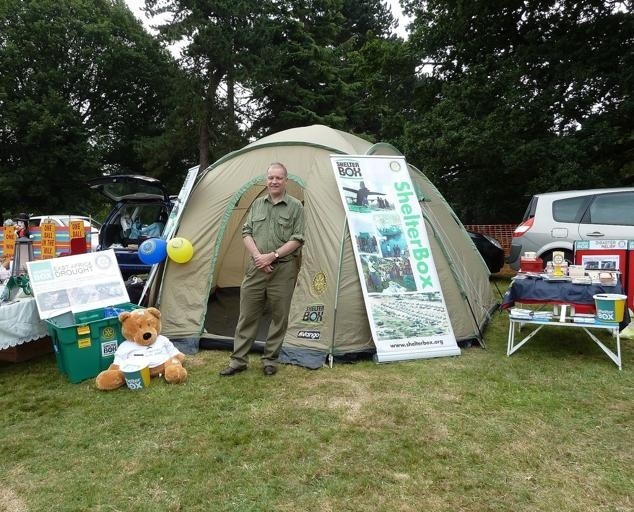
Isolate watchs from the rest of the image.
[273,250,280,262]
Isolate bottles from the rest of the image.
[11,237,33,285]
[545,261,587,281]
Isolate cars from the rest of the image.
[28,216,103,252]
[468,231,505,274]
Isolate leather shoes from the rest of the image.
[264,365,277,375]
[219,365,246,375]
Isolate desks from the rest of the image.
[506,271,625,370]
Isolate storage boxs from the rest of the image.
[43,303,143,383]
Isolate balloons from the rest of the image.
[135,238,169,265]
[165,236,194,264]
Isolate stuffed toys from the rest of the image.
[93,304,189,390]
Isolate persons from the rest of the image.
[356,230,413,292]
[219,161,307,376]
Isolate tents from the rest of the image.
[147,120,504,367]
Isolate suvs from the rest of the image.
[507,186,634,272]
[82,173,176,283]
